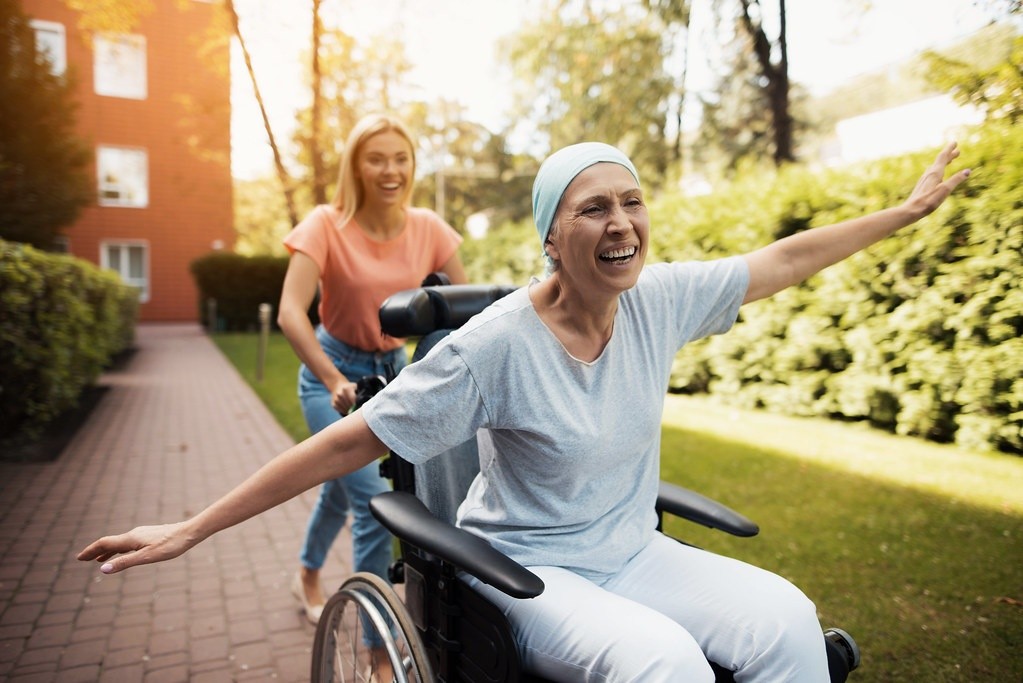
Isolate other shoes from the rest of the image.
[290,575,331,623]
[364,665,379,683]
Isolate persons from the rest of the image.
[82,141,972,683]
[275,113,469,683]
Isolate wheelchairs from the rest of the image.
[308,271,862,683]
